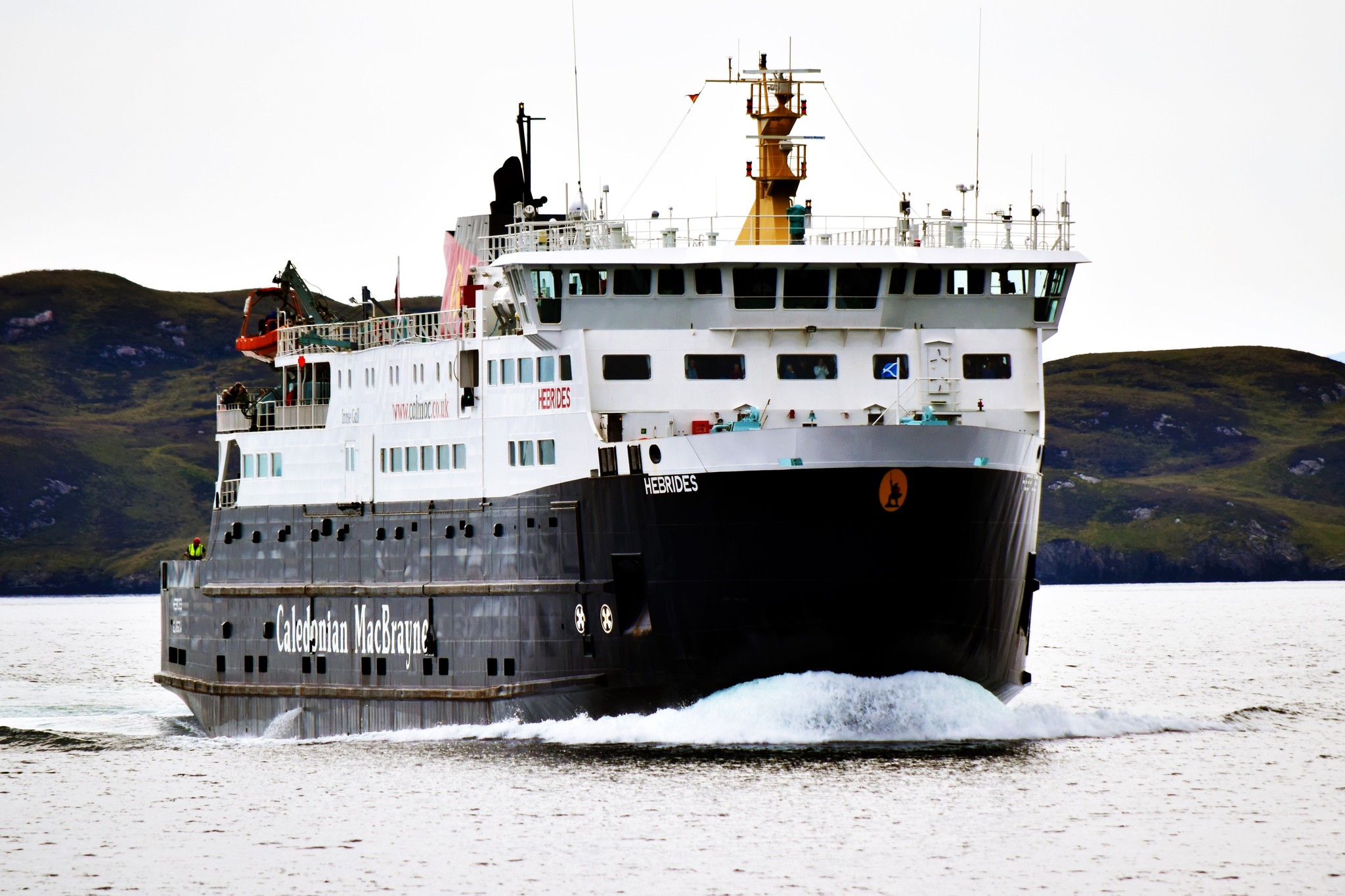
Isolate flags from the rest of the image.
[882,362,896,378]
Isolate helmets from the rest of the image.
[194,538,201,543]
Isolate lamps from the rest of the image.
[806,198,811,214]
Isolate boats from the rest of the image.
[235,287,306,364]
[151,5,1093,737]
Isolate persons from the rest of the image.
[230,382,248,410]
[220,389,233,410]
[729,363,743,380]
[287,385,298,406]
[814,359,829,379]
[687,359,698,379]
[965,356,1010,378]
[779,364,798,379]
